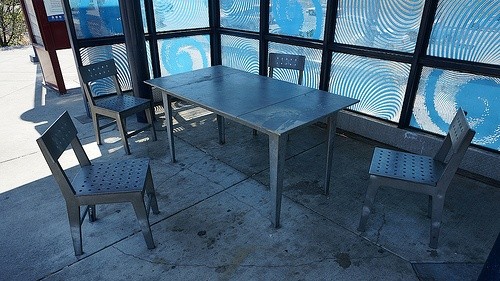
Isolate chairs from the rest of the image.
[78,58,158,157]
[36,109,161,258]
[361,110,478,252]
[253,52,306,142]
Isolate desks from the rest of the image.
[145,63,360,229]
